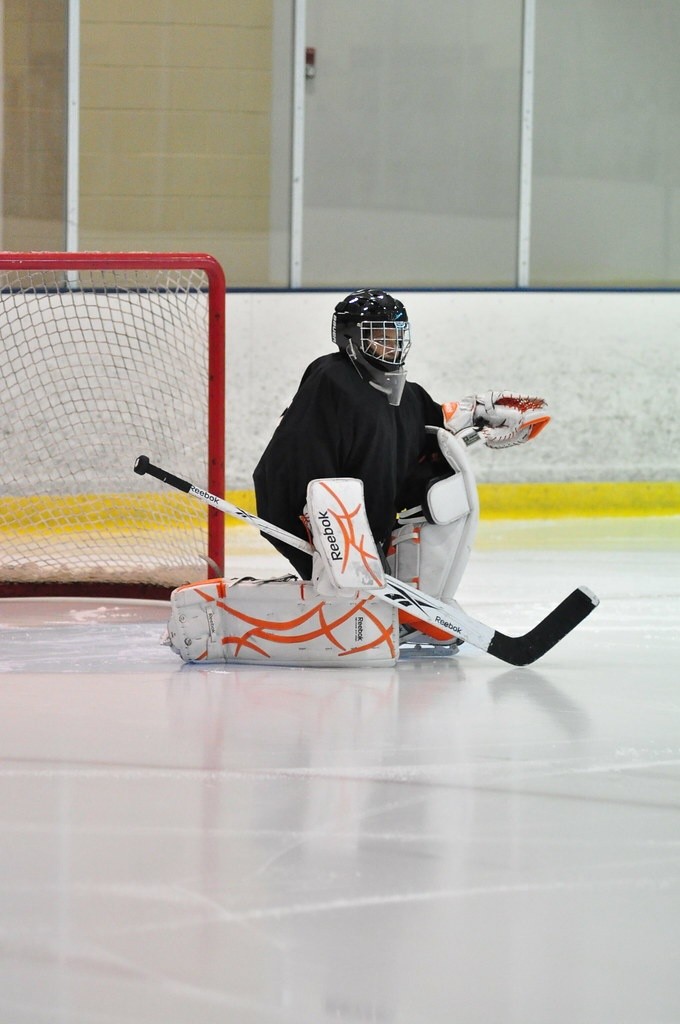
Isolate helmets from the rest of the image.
[331,289,408,373]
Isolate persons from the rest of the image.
[168,287,552,668]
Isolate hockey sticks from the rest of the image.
[133,454,602,667]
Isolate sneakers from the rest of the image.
[398,599,465,659]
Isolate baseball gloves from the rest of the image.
[441,389,552,454]
